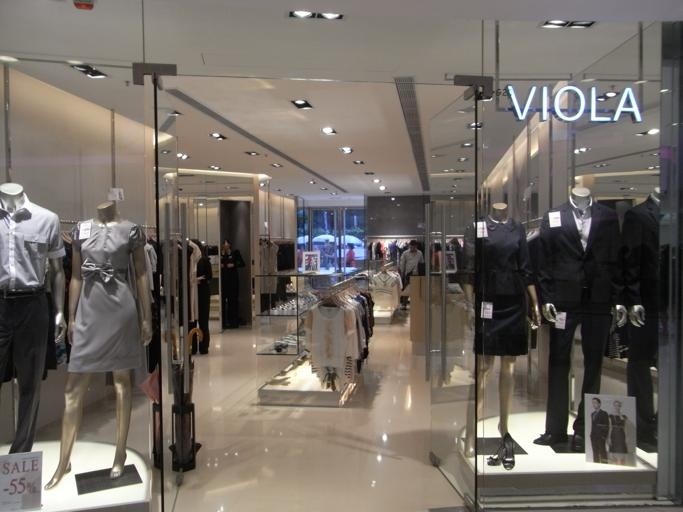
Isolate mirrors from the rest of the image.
[195,199,222,334]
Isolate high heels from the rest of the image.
[488,433,515,471]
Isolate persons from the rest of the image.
[42,201,150,490]
[621,187,662,452]
[462,204,540,458]
[219,239,245,329]
[297,243,305,267]
[0,183,67,450]
[318,239,336,268]
[607,400,629,465]
[399,240,424,310]
[532,187,630,451]
[589,397,611,461]
[345,243,354,267]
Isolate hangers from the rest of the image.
[318,288,345,307]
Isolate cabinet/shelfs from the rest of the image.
[255,268,317,354]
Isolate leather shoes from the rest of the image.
[534,432,567,445]
[572,433,585,451]
